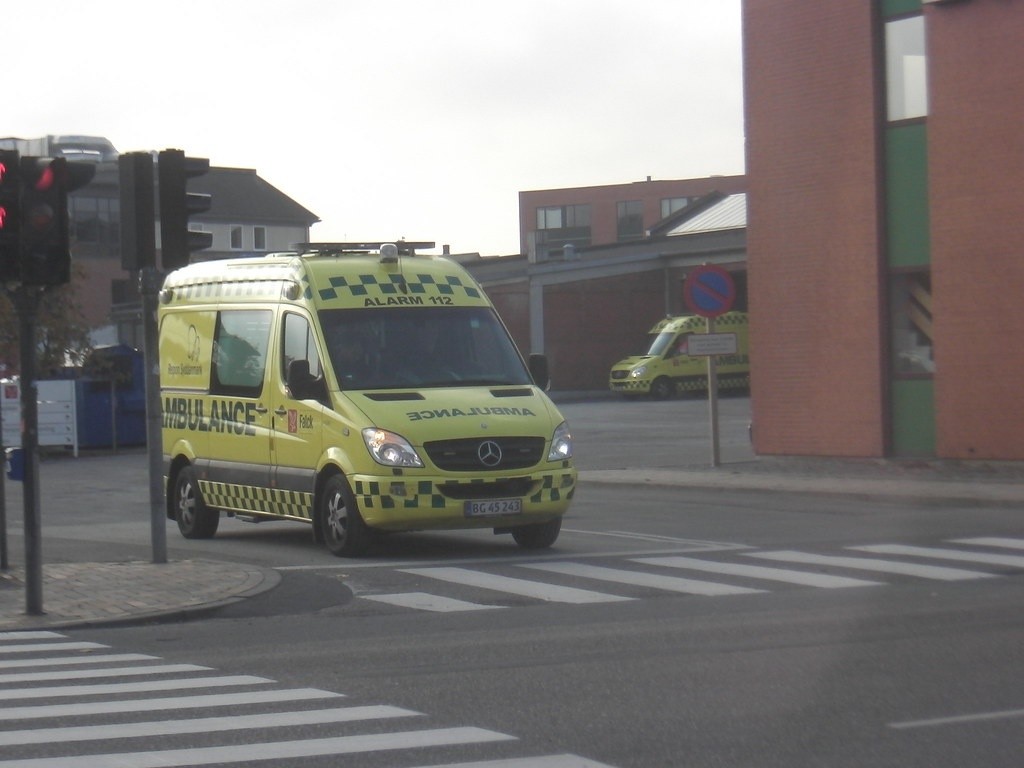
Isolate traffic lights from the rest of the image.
[0,145,96,288]
[157,150,212,267]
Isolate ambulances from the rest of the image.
[160,239,578,560]
[608,309,750,398]
